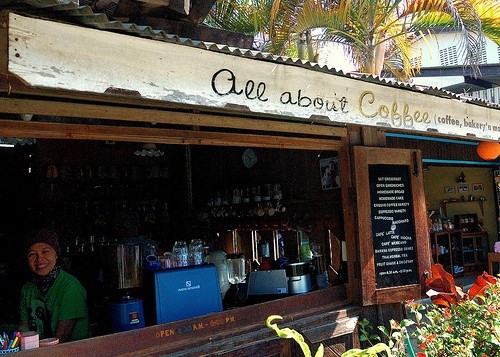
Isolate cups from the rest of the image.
[261,242,270,258]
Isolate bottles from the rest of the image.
[188,239,204,266]
[214,184,283,207]
[432,218,452,232]
[439,243,445,254]
[172,241,189,268]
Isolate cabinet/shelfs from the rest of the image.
[39,159,172,249]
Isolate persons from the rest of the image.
[18,230,89,344]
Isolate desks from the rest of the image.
[429,229,500,277]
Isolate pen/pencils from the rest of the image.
[0,332,21,350]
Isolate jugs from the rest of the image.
[225,252,251,284]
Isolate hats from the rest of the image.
[25,230,62,258]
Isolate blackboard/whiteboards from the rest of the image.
[350,145,434,306]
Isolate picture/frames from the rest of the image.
[445,183,484,193]
